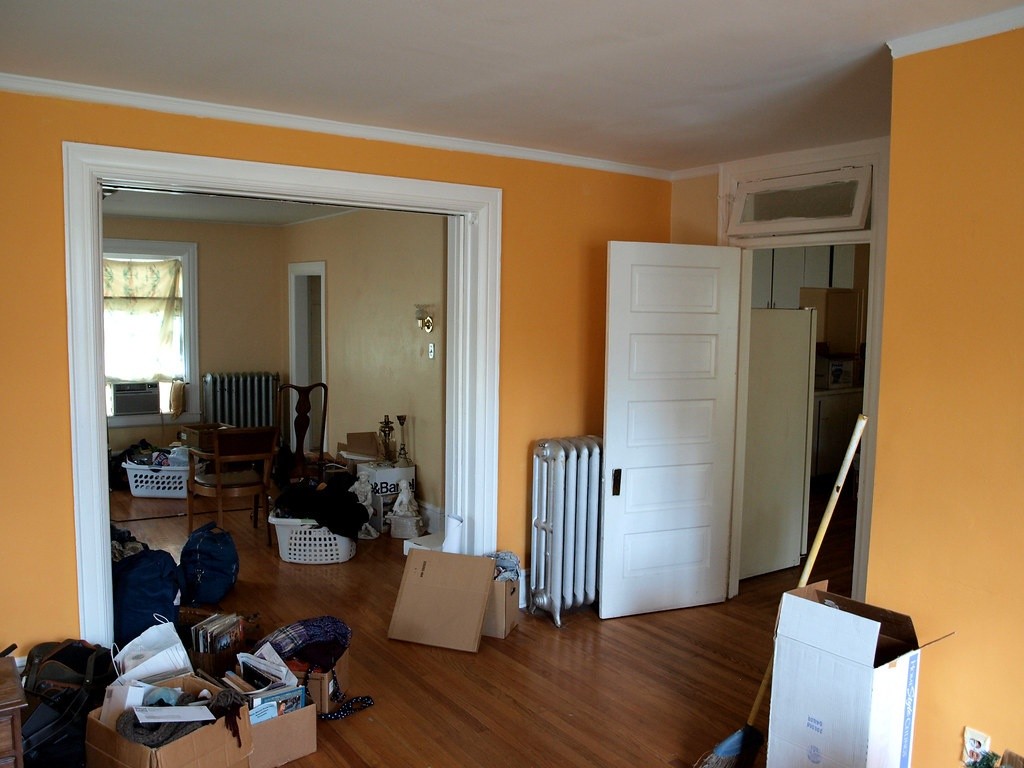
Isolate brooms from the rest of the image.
[685,411,868,768]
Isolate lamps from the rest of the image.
[415,302,433,332]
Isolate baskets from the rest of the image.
[122,460,210,499]
[268,508,355,565]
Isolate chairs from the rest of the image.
[184,425,280,550]
[248,381,329,530]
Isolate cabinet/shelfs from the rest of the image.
[370,492,415,534]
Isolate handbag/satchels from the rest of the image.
[178,521,239,607]
[110,613,196,680]
[112,548,177,639]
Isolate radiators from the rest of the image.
[527,435,604,626]
[201,370,281,428]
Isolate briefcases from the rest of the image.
[17,636,110,694]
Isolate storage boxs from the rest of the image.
[246,701,317,768]
[83,674,256,768]
[765,579,957,768]
[289,648,351,715]
[386,548,522,654]
[121,460,210,500]
[357,460,416,496]
[269,506,358,565]
[336,431,388,471]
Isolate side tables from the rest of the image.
[0,655,30,768]
